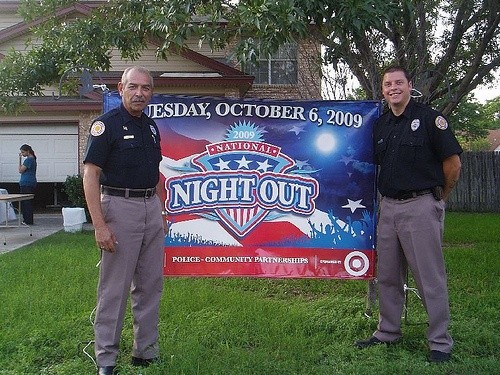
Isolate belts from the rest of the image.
[99,186,157,198]
[400,190,432,199]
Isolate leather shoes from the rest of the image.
[131,356,162,367]
[428,350,450,362]
[98,366,117,375]
[355,335,381,346]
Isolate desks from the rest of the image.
[0,194,35,228]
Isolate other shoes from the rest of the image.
[21,221,33,225]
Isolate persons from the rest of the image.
[84,66,164,375]
[354,65,464,362]
[18,144,37,226]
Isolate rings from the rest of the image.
[100,246,104,249]
